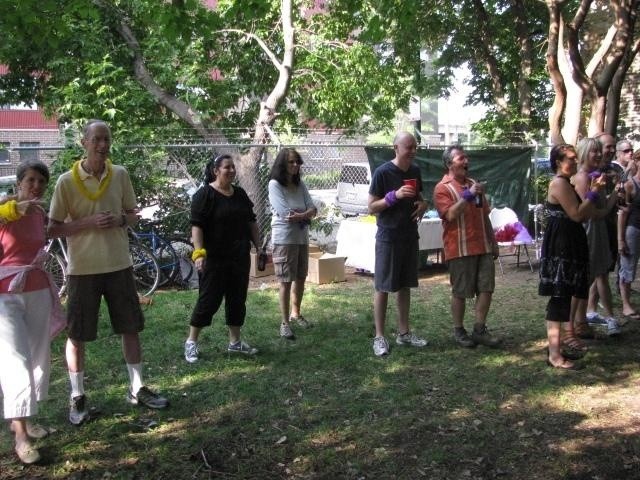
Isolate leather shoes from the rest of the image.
[546,356,583,370]
[547,347,583,359]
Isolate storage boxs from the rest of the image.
[303,252,348,286]
[308,242,321,252]
[249,248,275,279]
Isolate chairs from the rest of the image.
[486,204,535,276]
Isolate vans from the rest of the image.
[334,162,374,219]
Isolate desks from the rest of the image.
[336,214,447,275]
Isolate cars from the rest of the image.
[530,159,556,178]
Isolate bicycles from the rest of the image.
[42,218,196,300]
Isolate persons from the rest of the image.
[0,158,68,465]
[268,147,317,340]
[367,130,429,357]
[430,145,501,347]
[184,153,262,364]
[538,131,640,371]
[47,122,170,426]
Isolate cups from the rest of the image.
[404,179,417,196]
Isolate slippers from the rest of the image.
[622,312,640,320]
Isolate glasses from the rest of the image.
[615,148,634,153]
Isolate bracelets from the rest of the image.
[0,200,21,227]
[118,214,127,227]
[384,189,399,208]
[191,247,207,262]
[462,189,475,204]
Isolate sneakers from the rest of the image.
[127,386,170,411]
[472,322,501,346]
[184,338,199,364]
[451,327,475,347]
[9,422,48,440]
[280,321,293,337]
[68,394,89,427]
[606,316,622,336]
[290,313,311,328]
[395,332,429,347]
[585,311,609,326]
[15,442,40,466]
[373,336,391,357]
[227,341,260,356]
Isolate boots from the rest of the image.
[559,327,589,351]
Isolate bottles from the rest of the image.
[258,246,268,273]
[473,178,483,208]
[618,180,628,208]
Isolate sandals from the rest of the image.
[575,320,609,340]
